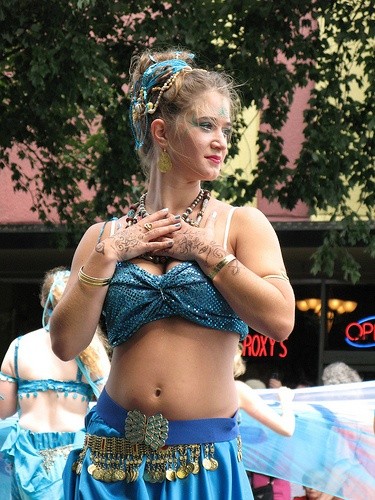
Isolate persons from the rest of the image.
[0,269,113,500]
[48,52,295,500]
[233,344,375,500]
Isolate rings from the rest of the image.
[144,221,152,231]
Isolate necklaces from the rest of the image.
[121,189,209,263]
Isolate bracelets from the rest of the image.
[206,254,238,281]
[78,265,113,286]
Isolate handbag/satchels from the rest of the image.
[252,477,274,500]
